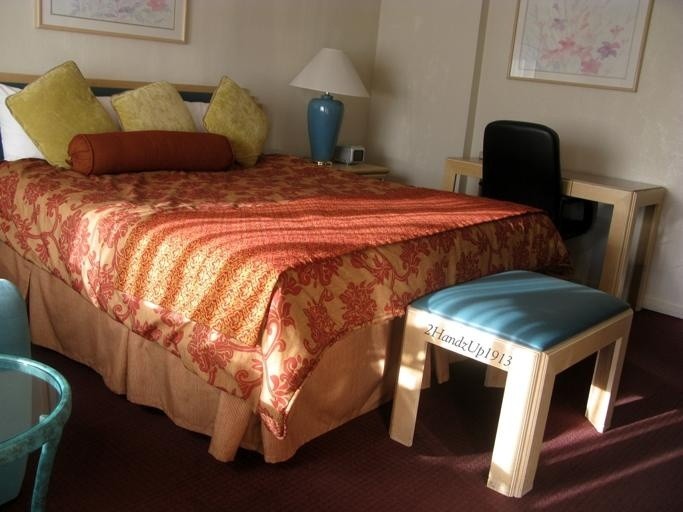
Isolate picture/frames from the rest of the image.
[36,0,189,44]
[507,0,655,93]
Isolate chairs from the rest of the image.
[479,121,596,269]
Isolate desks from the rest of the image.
[444,156,668,311]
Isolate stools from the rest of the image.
[389,269,634,497]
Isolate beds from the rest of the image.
[2,68,565,470]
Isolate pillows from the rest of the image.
[1,60,275,175]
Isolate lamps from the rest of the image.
[289,46,372,165]
